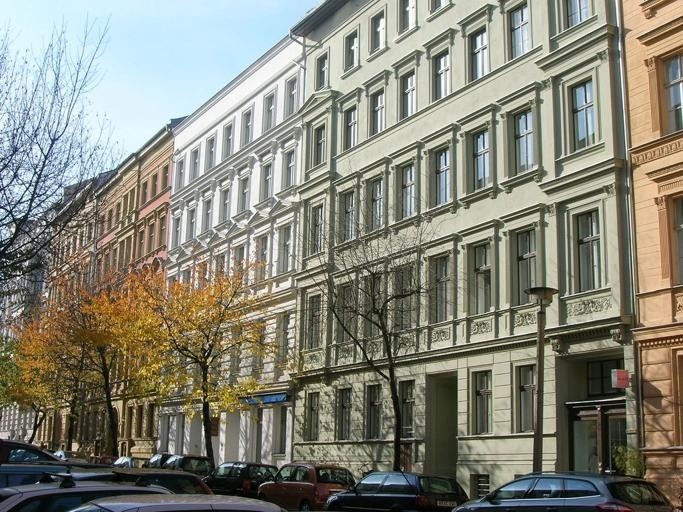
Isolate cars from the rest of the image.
[0,438,290,512]
[452,471,677,512]
[327,471,470,512]
[257,464,356,511]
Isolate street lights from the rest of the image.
[523,286,560,473]
